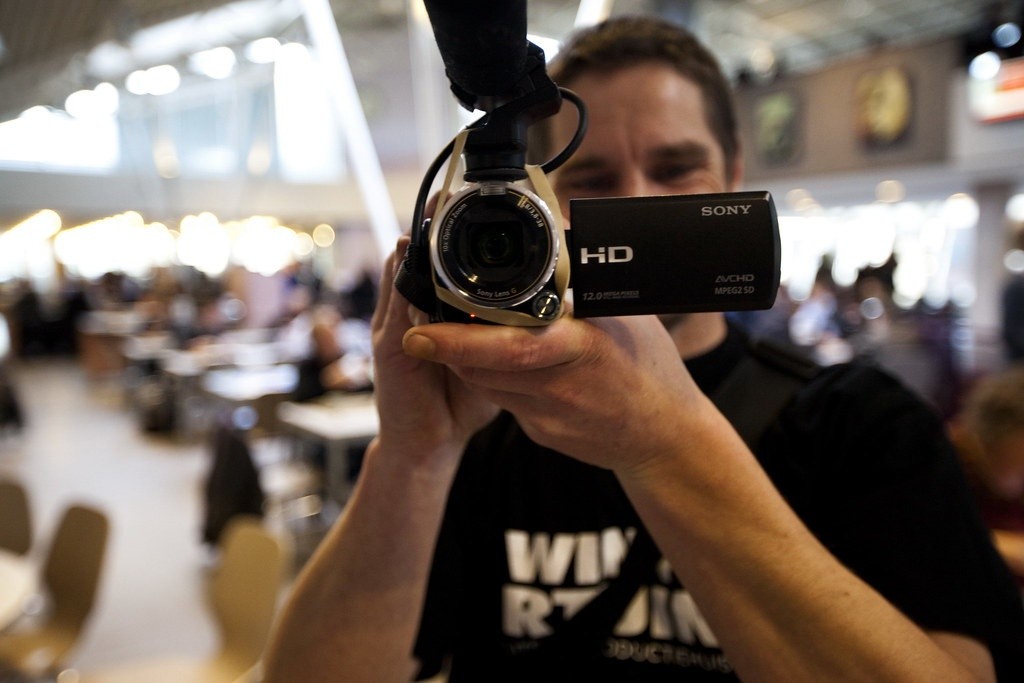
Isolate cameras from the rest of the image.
[396,43,782,327]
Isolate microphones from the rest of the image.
[423,0,529,109]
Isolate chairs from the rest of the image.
[0,426,288,683]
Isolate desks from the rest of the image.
[121,334,385,524]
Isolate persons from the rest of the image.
[257,16,1023,682]
[0,222,1022,537]
